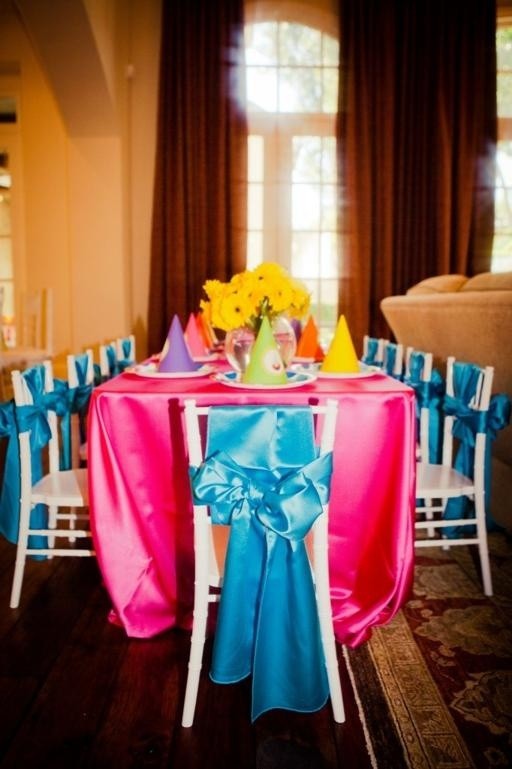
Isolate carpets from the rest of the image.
[0,556,512,769]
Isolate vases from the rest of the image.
[226,315,297,371]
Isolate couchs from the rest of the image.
[379,271,512,534]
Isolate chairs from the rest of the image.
[182,399,345,728]
[361,335,495,597]
[9,335,135,609]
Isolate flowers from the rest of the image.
[199,261,310,331]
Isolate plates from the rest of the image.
[209,371,318,390]
[133,362,219,379]
[292,363,381,379]
[194,352,218,362]
